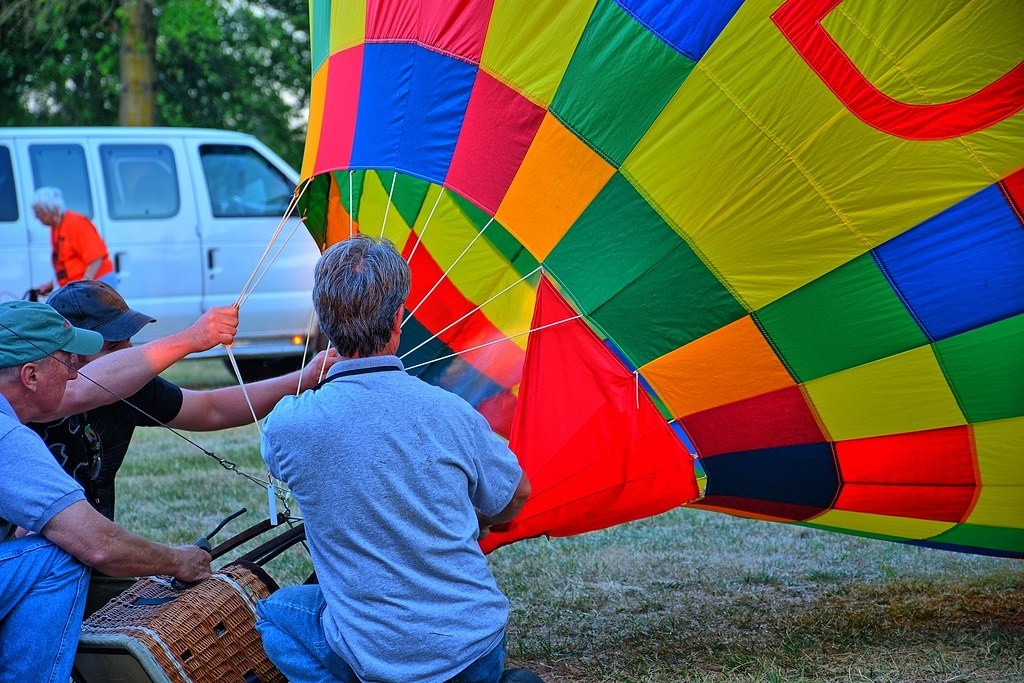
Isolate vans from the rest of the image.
[1,124,325,384]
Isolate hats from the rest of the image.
[0,300,104,369]
[45,280,157,342]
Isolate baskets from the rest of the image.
[71,560,290,683]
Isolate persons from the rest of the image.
[29,186,118,292]
[0,300,212,683]
[1,279,341,622]
[253,233,544,683]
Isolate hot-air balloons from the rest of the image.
[65,0,1024,682]
